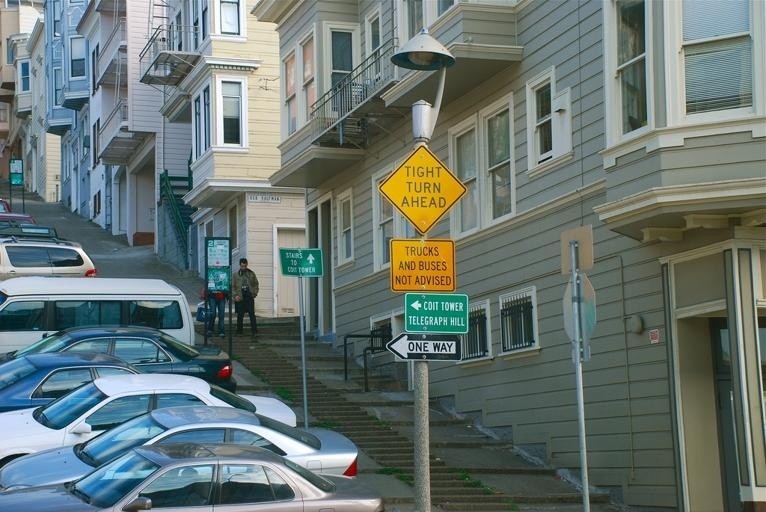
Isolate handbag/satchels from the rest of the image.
[199,287,207,300]
[197,303,212,322]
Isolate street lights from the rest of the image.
[384,23,458,510]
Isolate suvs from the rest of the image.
[0,198,58,239]
[1,235,96,281]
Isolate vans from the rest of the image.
[0,276,194,353]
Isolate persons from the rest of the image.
[200,281,228,337]
[231,257,260,339]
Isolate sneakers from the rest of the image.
[207,330,225,337]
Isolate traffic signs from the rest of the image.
[279,247,324,278]
[402,291,469,336]
[386,332,462,362]
[377,145,466,234]
[387,235,456,295]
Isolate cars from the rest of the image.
[0,373,298,466]
[0,350,144,414]
[0,442,383,512]
[0,323,238,394]
[0,404,361,492]
